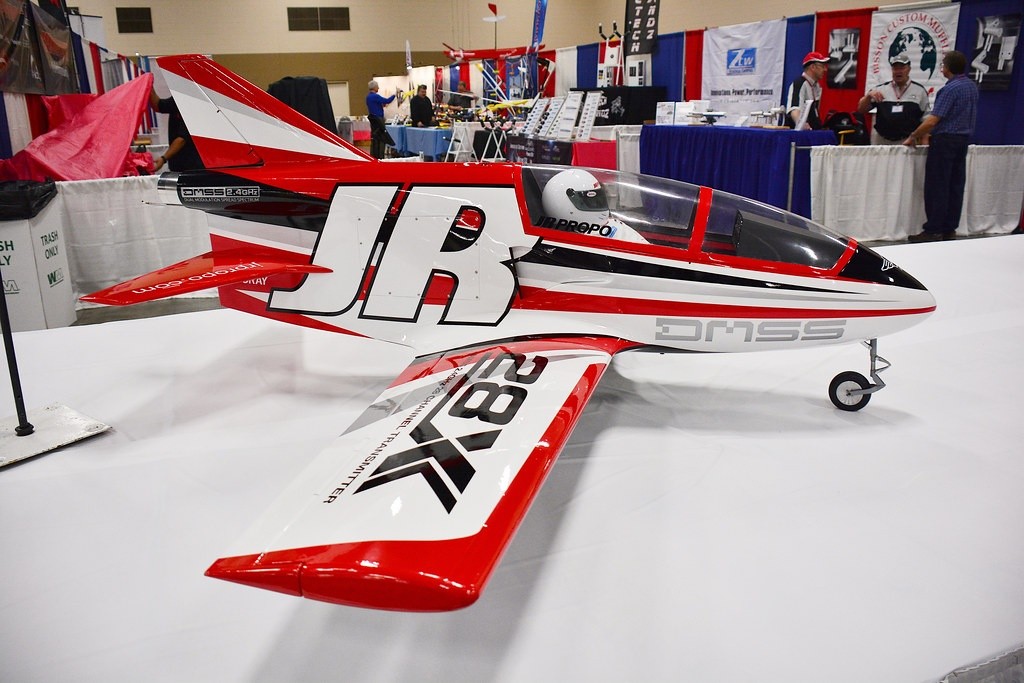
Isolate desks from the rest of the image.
[406,128,454,162]
[386,124,413,157]
[639,124,834,234]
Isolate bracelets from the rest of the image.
[161,156,167,163]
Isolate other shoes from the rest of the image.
[908,229,945,243]
[942,230,956,240]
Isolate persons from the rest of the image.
[858,54,930,145]
[366,80,395,158]
[150,88,205,173]
[410,85,435,127]
[902,51,979,242]
[543,170,648,244]
[448,82,479,111]
[784,52,830,130]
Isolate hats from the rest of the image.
[892,54,911,64]
[802,52,830,65]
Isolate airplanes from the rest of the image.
[78,48,936,615]
[685,110,727,123]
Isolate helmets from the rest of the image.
[542,167,609,230]
